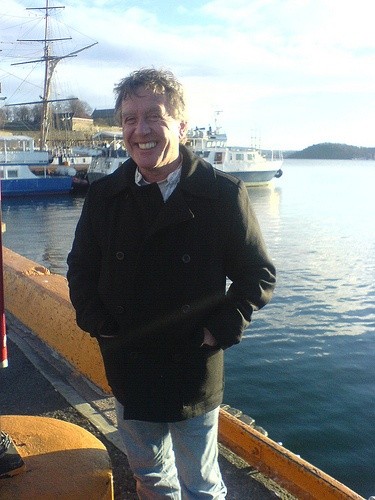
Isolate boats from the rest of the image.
[87,111,282,189]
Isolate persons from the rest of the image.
[67,71,276,500]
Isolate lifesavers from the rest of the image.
[274,169,283,178]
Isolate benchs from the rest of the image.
[0,415,115,500]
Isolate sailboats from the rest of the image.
[0,0,103,198]
